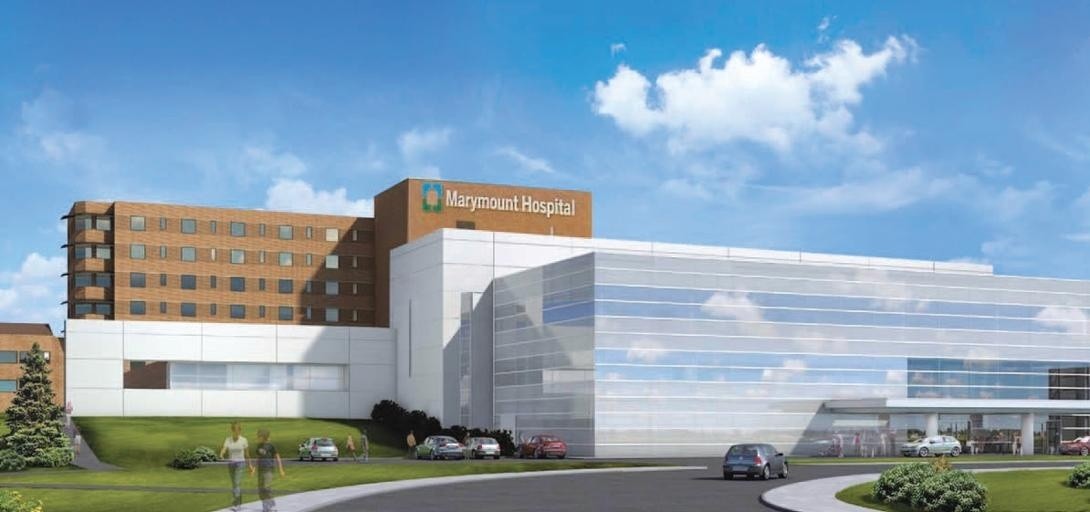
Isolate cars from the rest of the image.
[295,435,341,463]
[807,438,841,458]
[1059,435,1090,457]
[414,433,466,460]
[525,433,567,458]
[722,441,791,481]
[462,436,501,459]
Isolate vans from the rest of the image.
[898,434,966,459]
